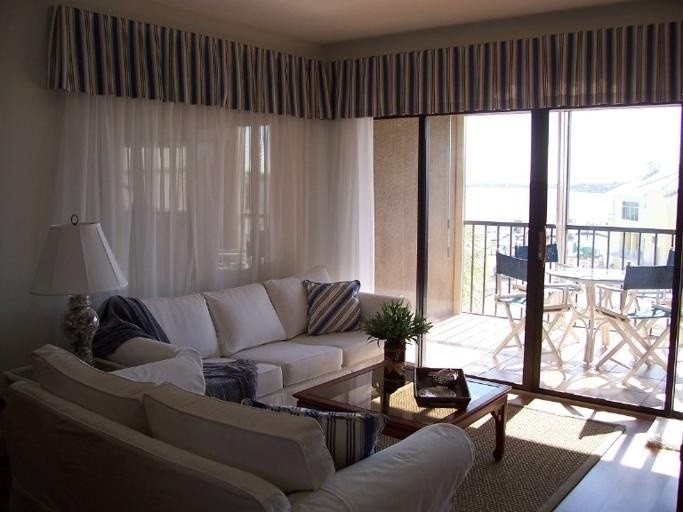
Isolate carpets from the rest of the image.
[375,402,626,510]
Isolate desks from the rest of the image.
[3,354,125,384]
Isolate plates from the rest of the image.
[415,386,456,399]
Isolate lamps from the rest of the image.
[30,213,130,369]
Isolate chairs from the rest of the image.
[492,241,682,387]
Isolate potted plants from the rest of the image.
[362,296,432,378]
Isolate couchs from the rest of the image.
[0,344,478,511]
[98,265,416,405]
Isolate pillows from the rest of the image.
[303,279,362,335]
[237,394,390,469]
[108,348,206,396]
